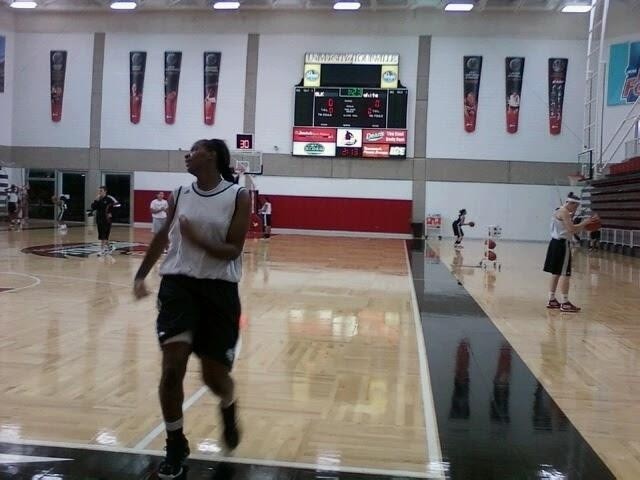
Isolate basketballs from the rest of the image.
[586,216,601,231]
[482,236,498,262]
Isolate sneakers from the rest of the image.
[105,244,115,255]
[216,402,242,451]
[155,433,190,479]
[97,245,104,255]
[559,300,582,313]
[545,296,561,308]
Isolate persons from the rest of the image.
[258,196,272,239]
[4,186,20,226]
[91,186,121,256]
[451,209,470,248]
[542,192,595,313]
[52,190,69,230]
[588,207,601,251]
[149,191,170,254]
[132,139,251,480]
